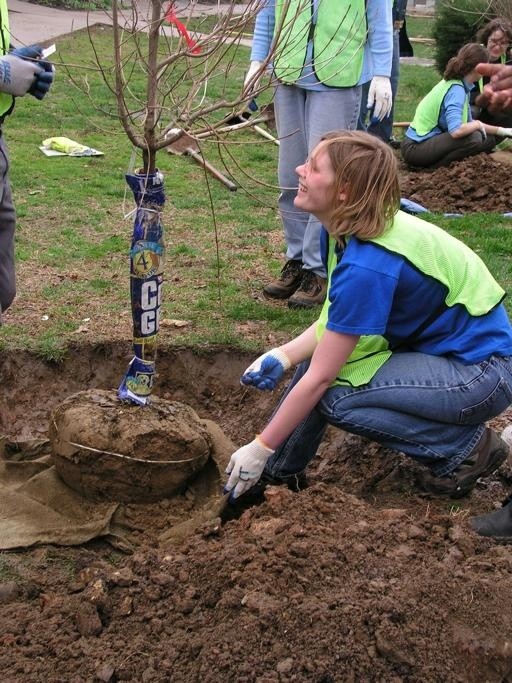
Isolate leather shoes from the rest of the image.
[244,61,262,99]
[224,434,275,503]
[366,76,392,123]
[496,127,512,137]
[0,43,56,101]
[475,120,487,140]
[240,348,291,391]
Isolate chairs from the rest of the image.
[263,259,328,310]
[418,428,510,500]
[467,502,512,541]
[220,474,309,525]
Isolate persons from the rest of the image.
[225,130,512,499]
[475,63,512,113]
[0,0,54,316]
[401,44,512,167]
[359,1,414,142]
[468,19,512,126]
[246,0,392,306]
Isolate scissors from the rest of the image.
[224,110,280,145]
[164,128,237,191]
